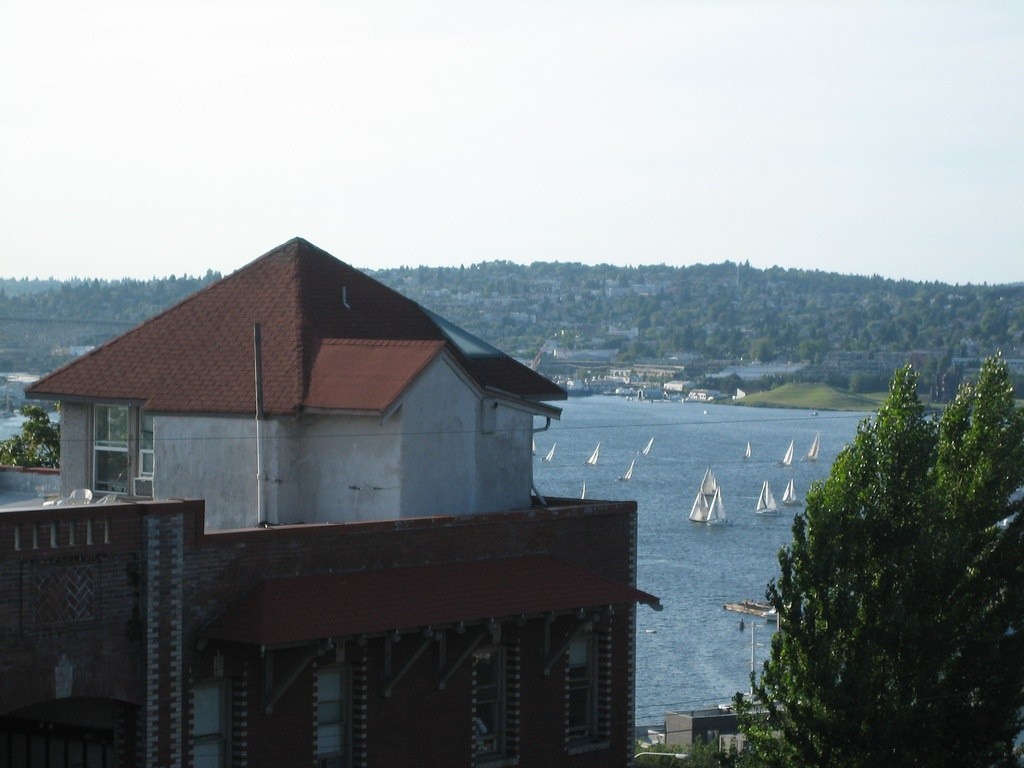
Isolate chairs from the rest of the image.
[53,488,93,507]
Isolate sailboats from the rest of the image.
[743,442,750,459]
[706,485,732,526]
[688,490,710,523]
[781,479,802,505]
[700,462,716,495]
[614,459,635,482]
[637,437,654,456]
[772,440,794,467]
[800,434,820,462]
[754,480,784,517]
[541,442,557,461]
[585,442,600,464]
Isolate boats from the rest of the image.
[725,600,805,621]
